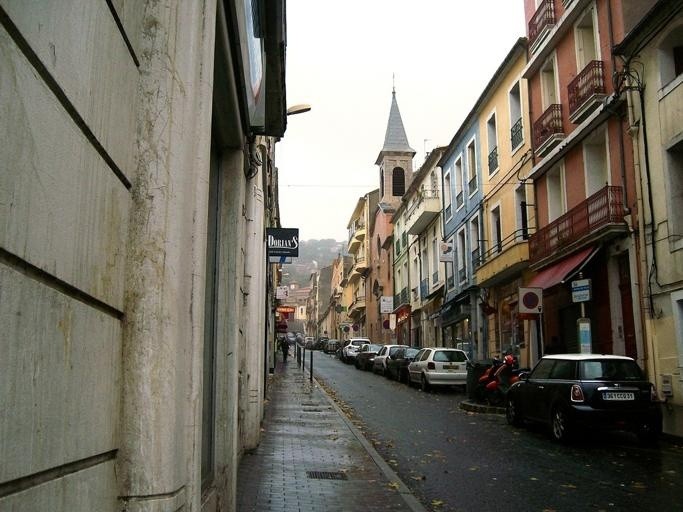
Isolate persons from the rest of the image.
[279,337,291,363]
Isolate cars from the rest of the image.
[285,330,470,393]
[502,352,667,445]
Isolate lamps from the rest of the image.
[287,104,311,115]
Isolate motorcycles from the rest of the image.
[481,352,531,397]
[470,354,531,401]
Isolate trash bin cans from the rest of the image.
[466,359,495,404]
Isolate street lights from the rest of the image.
[286,104,316,118]
